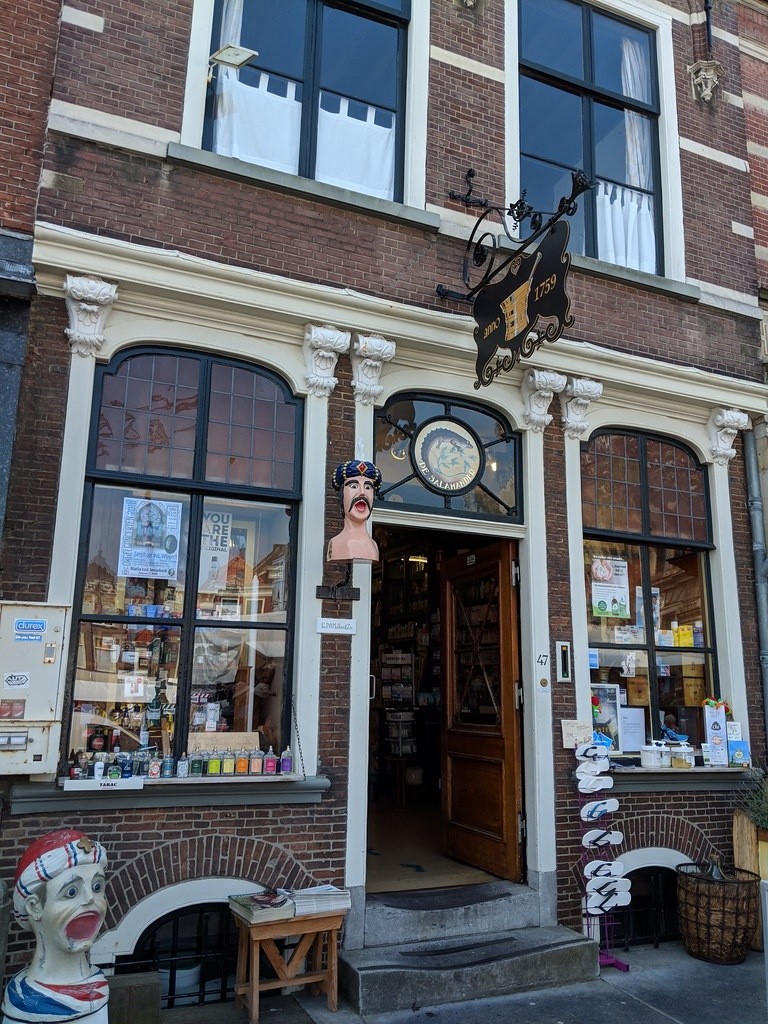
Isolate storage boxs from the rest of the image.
[658,652,704,677]
[659,677,706,707]
[675,862,761,965]
[703,704,750,768]
[627,675,650,706]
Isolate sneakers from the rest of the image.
[647,723,689,744]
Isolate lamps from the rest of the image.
[206,44,259,84]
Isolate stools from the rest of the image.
[231,910,347,1024]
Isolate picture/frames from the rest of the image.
[590,683,623,755]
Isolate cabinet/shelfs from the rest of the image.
[665,707,705,766]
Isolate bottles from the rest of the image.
[235,746,249,776]
[208,556,219,591]
[149,752,161,778]
[207,746,221,776]
[263,746,277,776]
[249,746,261,776]
[694,621,704,646]
[161,748,173,778]
[114,738,120,752]
[140,750,150,774]
[190,747,204,776]
[177,751,189,777]
[671,621,679,646]
[69,749,140,779]
[280,746,295,773]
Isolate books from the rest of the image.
[228,884,352,924]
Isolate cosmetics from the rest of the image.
[68,626,293,780]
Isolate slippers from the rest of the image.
[587,890,631,915]
[575,745,607,761]
[585,877,631,896]
[577,776,613,794]
[584,861,624,880]
[575,758,610,780]
[580,799,619,822]
[582,830,623,848]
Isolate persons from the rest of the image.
[640,596,659,644]
[1,830,110,1024]
[165,536,175,554]
[248,893,287,909]
[327,460,379,563]
